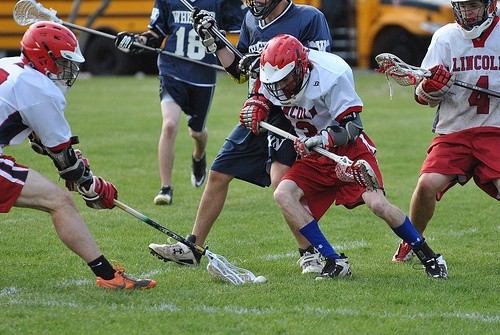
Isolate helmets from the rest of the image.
[451,0,497,39]
[258,34,311,105]
[20,20,86,88]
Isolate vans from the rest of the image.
[0,0,456,77]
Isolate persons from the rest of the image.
[148,0,332,273]
[114,0,248,204]
[239,34,448,279]
[0,21,156,289]
[393,0,500,261]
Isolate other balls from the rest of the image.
[253,275,266,283]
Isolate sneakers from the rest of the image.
[412,253,448,279]
[298,245,323,274]
[191,147,206,187]
[94,267,156,291]
[391,242,416,262]
[149,235,210,268]
[316,253,352,280]
[154,186,172,204]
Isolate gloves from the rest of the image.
[113,31,145,54]
[76,176,118,210]
[238,53,261,79]
[64,149,88,192]
[414,64,456,107]
[293,126,349,159]
[239,93,271,136]
[190,6,227,55]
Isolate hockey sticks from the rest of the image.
[12,0,260,79]
[373,53,500,99]
[112,197,267,284]
[260,123,380,193]
